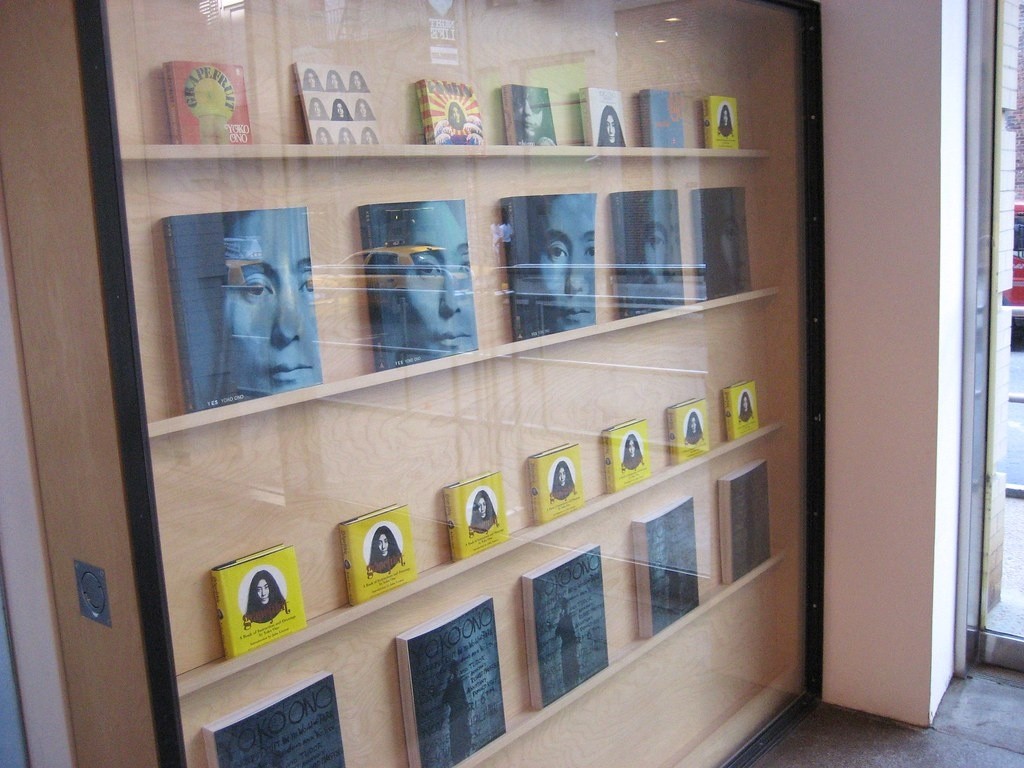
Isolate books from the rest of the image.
[163,60,739,148]
[201,382,770,768]
[163,187,750,435]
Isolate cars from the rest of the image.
[1011,205,1024,321]
[315,244,472,319]
[223,257,286,326]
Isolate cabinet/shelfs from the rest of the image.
[0,0,826,768]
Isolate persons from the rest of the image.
[225,209,322,392]
[553,461,574,500]
[472,490,497,532]
[389,202,477,355]
[739,392,753,421]
[515,86,556,147]
[635,191,684,307]
[623,434,643,468]
[686,412,703,445]
[719,106,732,129]
[597,105,626,147]
[302,69,379,144]
[532,194,595,334]
[246,569,284,622]
[369,526,401,573]
